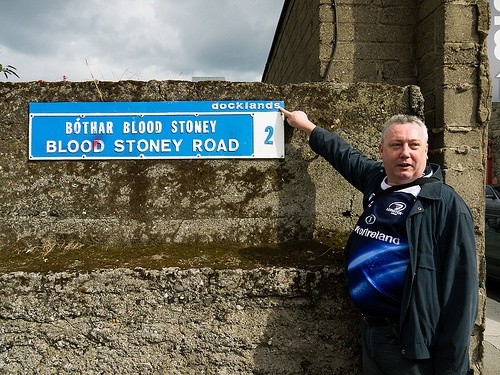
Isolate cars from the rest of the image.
[485,184,500,223]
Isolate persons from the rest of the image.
[280,106,478,375]
